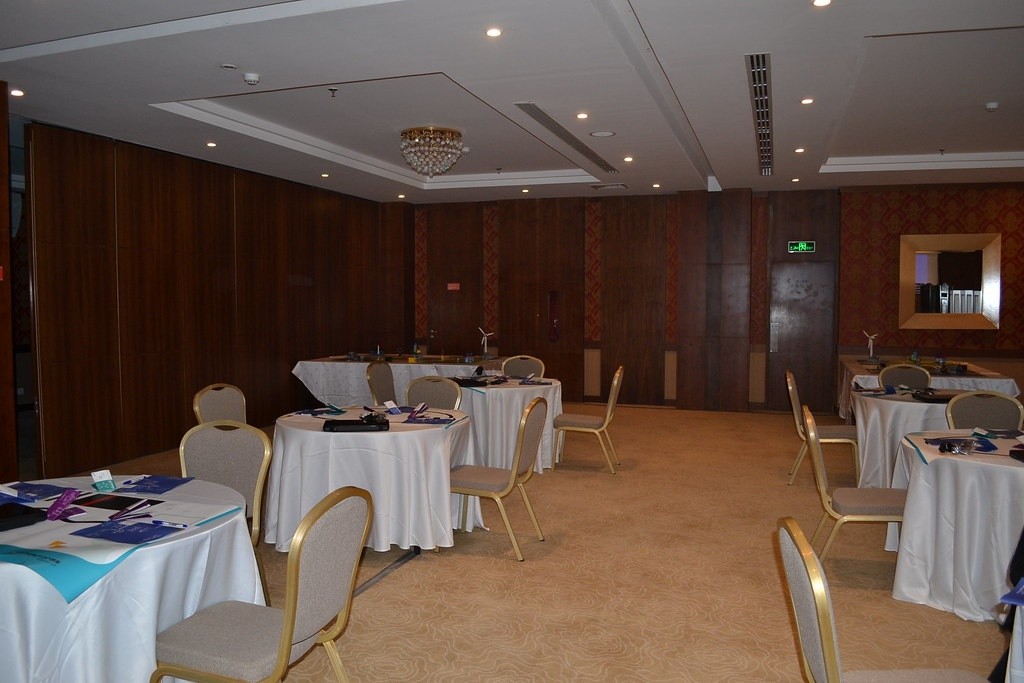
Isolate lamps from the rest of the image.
[399,126,464,177]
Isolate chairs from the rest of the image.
[449,396,548,561]
[405,376,463,411]
[945,389,1024,430]
[502,355,546,378]
[776,516,992,683]
[150,486,374,683]
[878,364,931,390]
[192,382,248,428]
[550,365,625,475]
[784,368,861,488]
[368,360,400,408]
[802,405,907,565]
[179,420,274,546]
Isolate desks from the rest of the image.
[266,406,491,553]
[835,354,1022,425]
[290,353,515,409]
[0,475,268,683]
[457,374,563,474]
[851,389,973,554]
[885,429,1024,624]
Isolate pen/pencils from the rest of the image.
[433,418,455,420]
[123,476,146,485]
[377,345,379,355]
[153,520,187,527]
[862,392,886,394]
